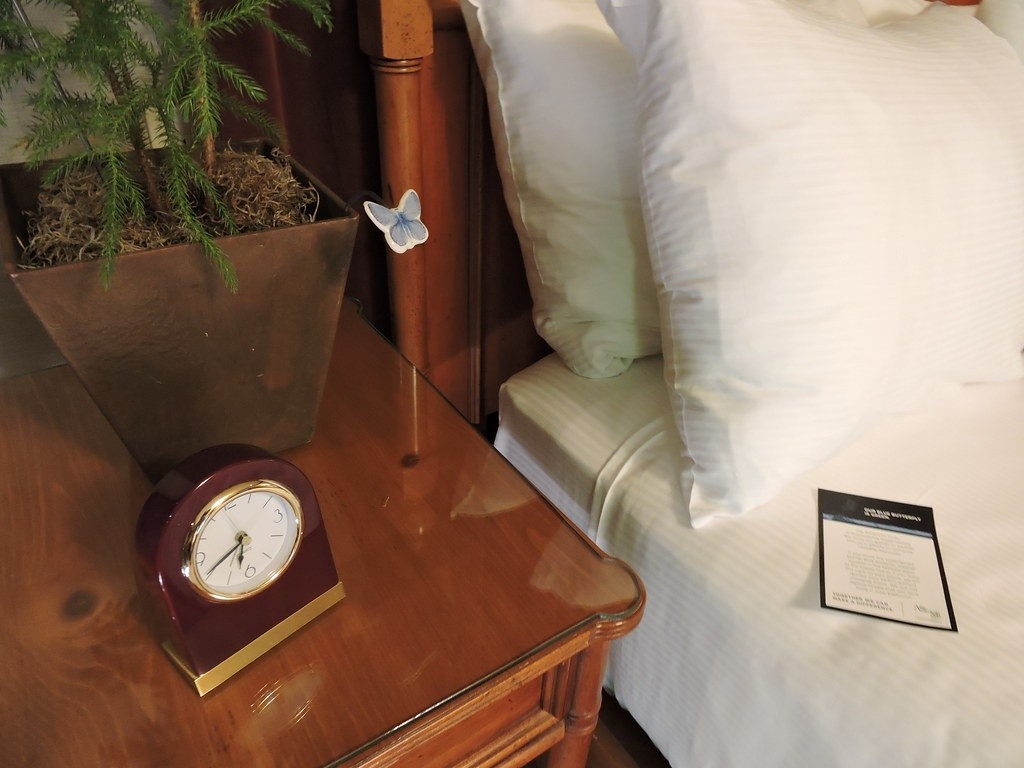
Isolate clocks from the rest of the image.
[135,443,345,697]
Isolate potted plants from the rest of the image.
[1,0,361,484]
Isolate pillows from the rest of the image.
[597,0,1024,529]
[455,0,658,380]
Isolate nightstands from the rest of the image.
[0,290,647,768]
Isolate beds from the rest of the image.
[357,0,1024,768]
